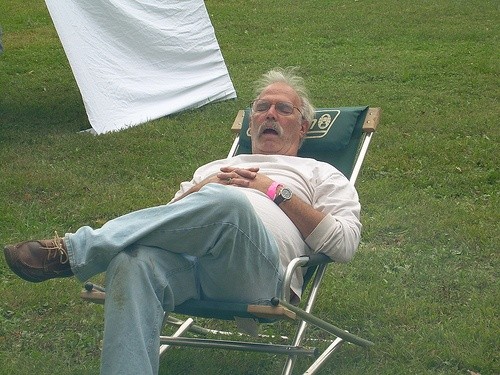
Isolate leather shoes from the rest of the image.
[3,230,74,283]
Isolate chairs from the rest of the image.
[79,106,382,375]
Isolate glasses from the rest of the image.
[248,98,306,120]
